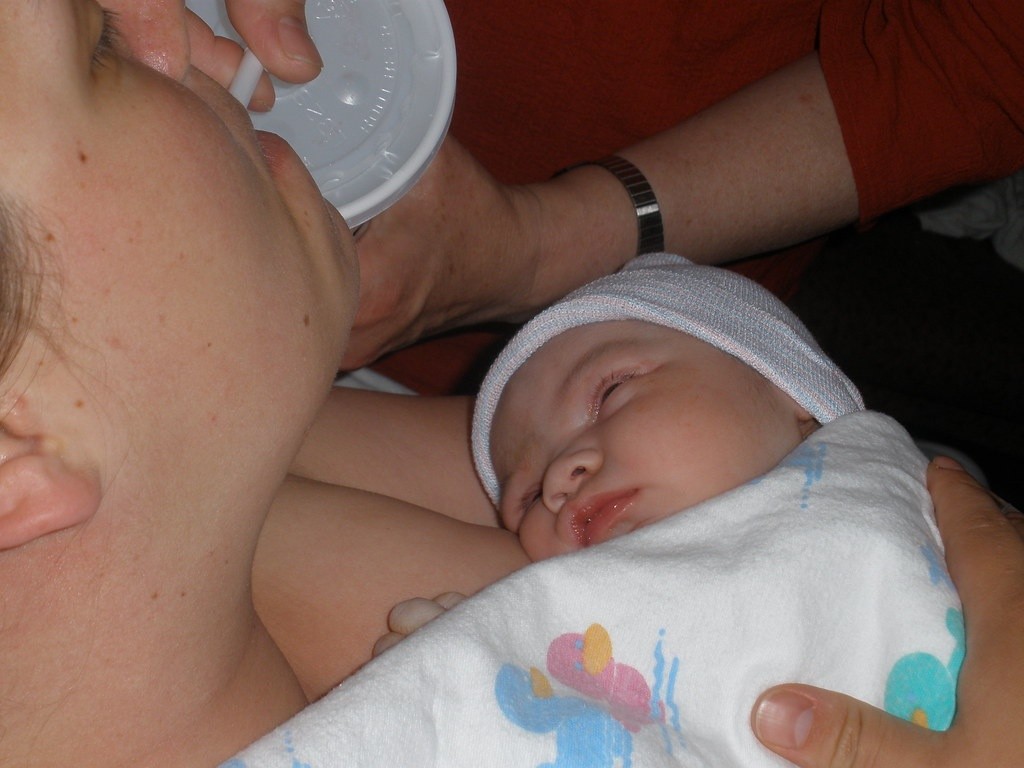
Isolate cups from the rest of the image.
[182,0,459,232]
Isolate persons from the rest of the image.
[0,0,1024,768]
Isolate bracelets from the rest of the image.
[548,155,665,256]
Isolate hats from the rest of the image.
[471,252,866,510]
